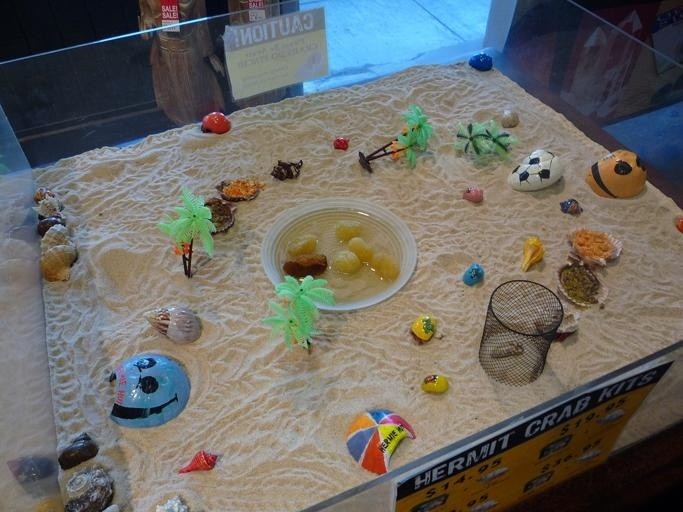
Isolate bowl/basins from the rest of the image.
[263,199,417,311]
